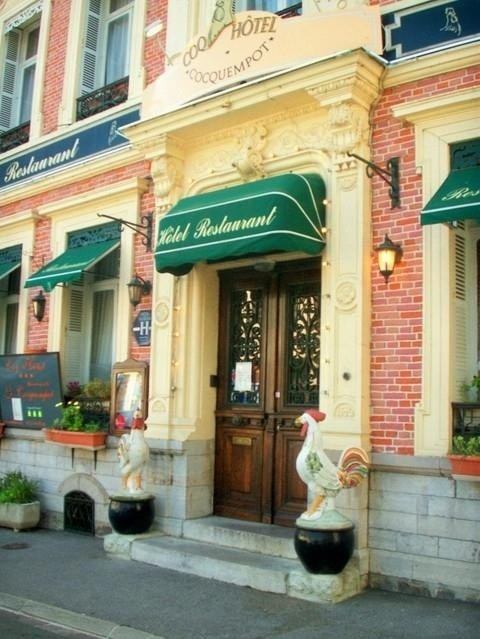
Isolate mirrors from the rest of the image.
[108,356,148,441]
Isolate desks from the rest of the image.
[452,401,480,438]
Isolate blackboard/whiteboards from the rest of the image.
[0,352,67,428]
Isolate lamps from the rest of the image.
[125,271,153,310]
[31,288,49,323]
[373,229,405,283]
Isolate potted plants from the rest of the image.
[459,375,480,402]
[43,395,108,449]
[1,466,43,536]
[447,431,480,477]
